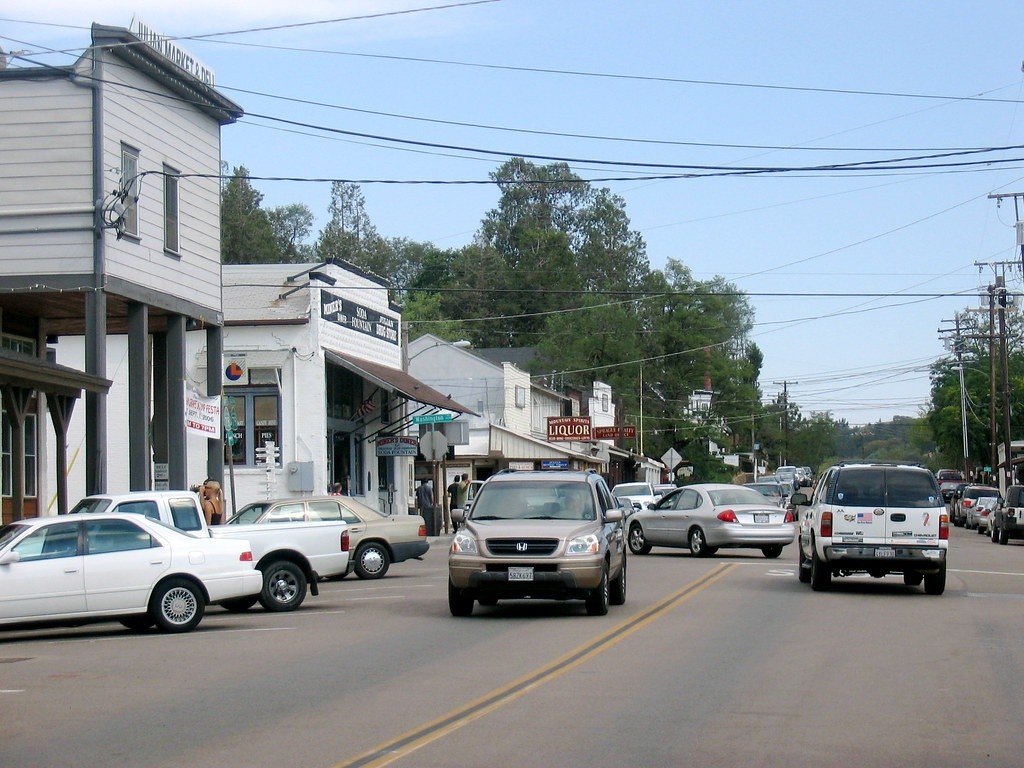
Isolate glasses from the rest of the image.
[205,486,212,490]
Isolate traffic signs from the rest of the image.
[255,441,282,494]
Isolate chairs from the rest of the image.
[496,488,528,517]
[836,484,860,504]
[543,502,560,517]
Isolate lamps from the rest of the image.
[279,271,337,300]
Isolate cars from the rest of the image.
[652,485,680,507]
[935,469,1024,546]
[741,465,816,522]
[616,498,640,521]
[626,483,797,559]
[611,483,663,513]
[225,495,430,581]
[0,512,264,633]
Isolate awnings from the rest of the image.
[324,348,481,444]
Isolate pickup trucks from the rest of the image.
[13,490,355,614]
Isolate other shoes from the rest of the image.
[453,530,457,533]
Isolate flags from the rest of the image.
[354,400,375,418]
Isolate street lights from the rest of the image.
[951,367,998,487]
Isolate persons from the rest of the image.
[199,478,225,525]
[329,482,342,496]
[416,479,433,509]
[447,474,470,533]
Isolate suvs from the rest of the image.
[448,468,630,617]
[790,461,951,596]
